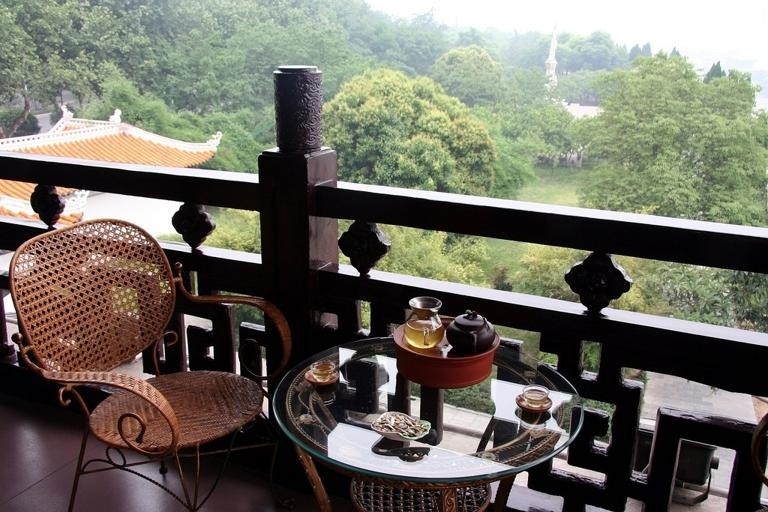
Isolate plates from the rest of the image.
[515,396,556,411]
[304,371,340,385]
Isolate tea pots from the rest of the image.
[404,297,444,350]
[445,309,494,353]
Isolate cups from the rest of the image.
[311,359,335,382]
[522,384,549,406]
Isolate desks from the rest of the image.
[274,338,584,511]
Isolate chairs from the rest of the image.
[6,217,330,511]
